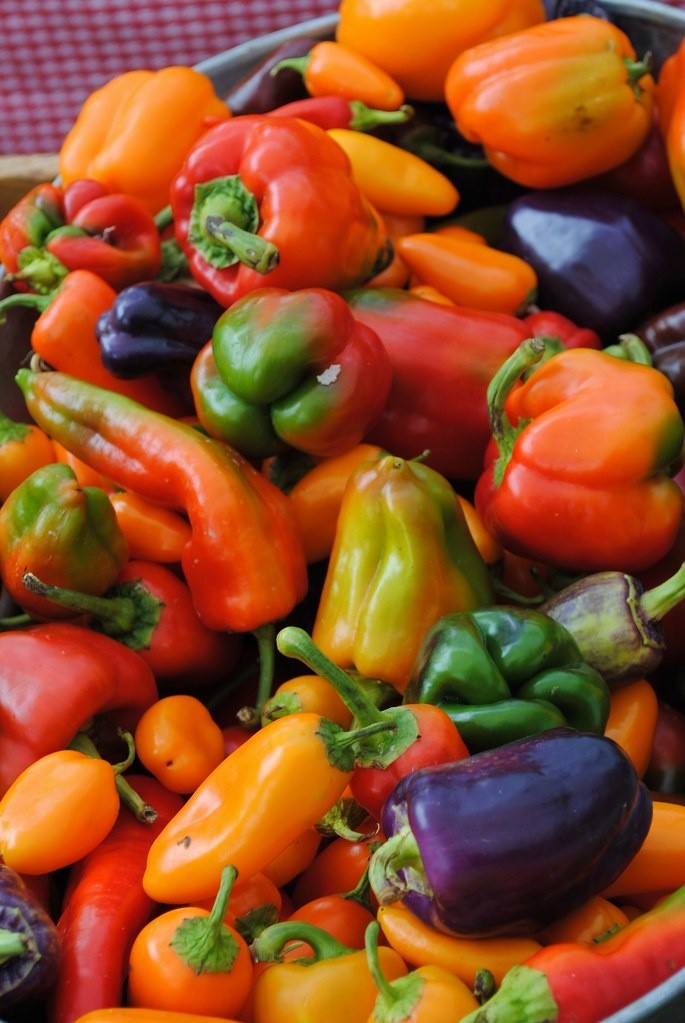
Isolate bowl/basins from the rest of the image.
[0,0,685,1023]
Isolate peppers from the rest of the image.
[0,0,685,1023]
